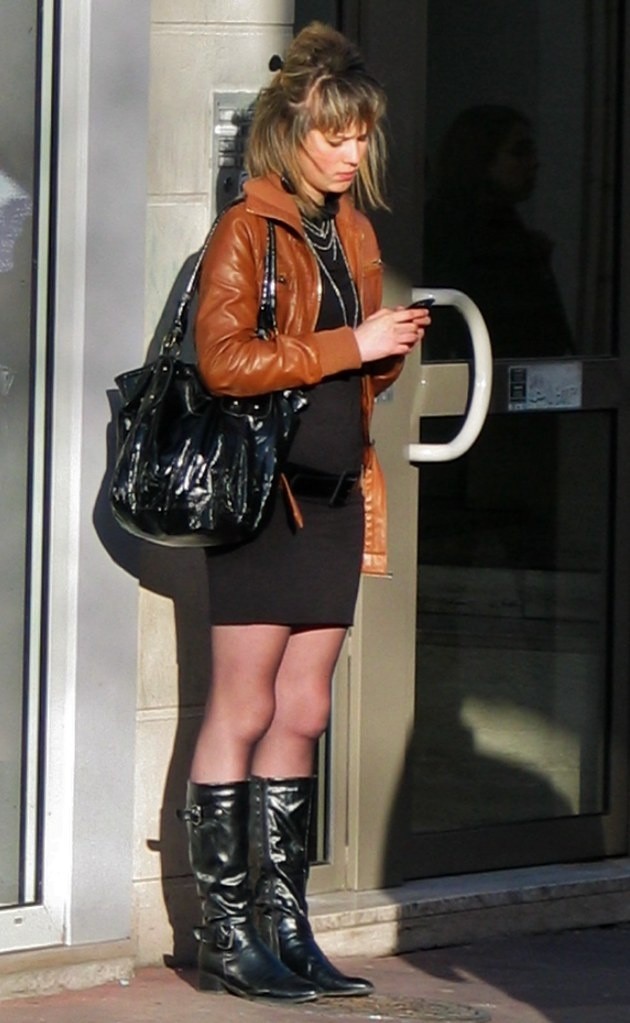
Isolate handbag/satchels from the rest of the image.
[108,195,308,548]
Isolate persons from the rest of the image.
[180,18,430,1005]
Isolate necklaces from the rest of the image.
[299,213,358,330]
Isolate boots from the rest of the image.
[242,773,376,995]
[179,778,326,1006]
